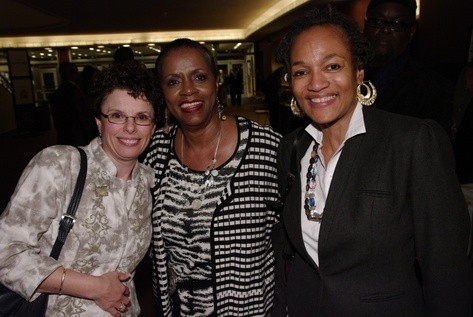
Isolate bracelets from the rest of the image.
[58,268,66,295]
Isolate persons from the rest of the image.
[269,0,473,317]
[142,38,283,317]
[0,46,162,317]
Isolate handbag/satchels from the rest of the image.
[1,144,87,317]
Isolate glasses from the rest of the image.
[99,110,157,126]
[366,12,412,36]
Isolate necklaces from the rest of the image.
[304,143,323,223]
[182,119,222,210]
